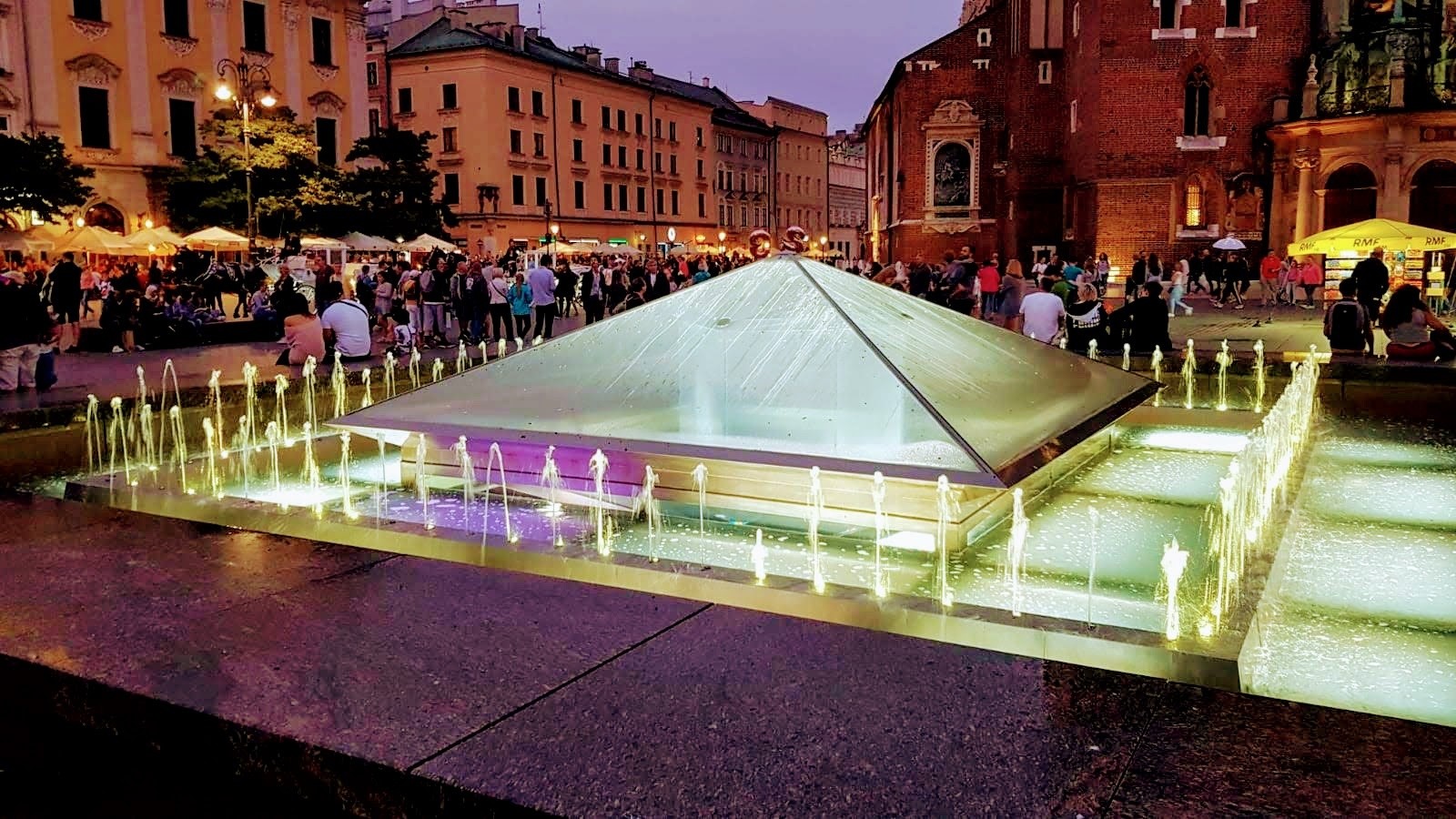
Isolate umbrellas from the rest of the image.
[1287,218,1456,255]
[0,222,779,266]
[1212,239,1246,249]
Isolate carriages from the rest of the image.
[173,246,350,320]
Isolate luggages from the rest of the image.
[1299,304,1314,309]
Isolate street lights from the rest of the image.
[214,57,282,265]
[550,224,559,251]
[718,232,726,258]
[820,236,827,263]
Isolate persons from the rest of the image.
[0,247,1456,391]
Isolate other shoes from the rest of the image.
[457,332,491,346]
[1185,307,1193,315]
[1168,313,1175,317]
[412,335,456,350]
[1209,296,1245,309]
[112,346,124,353]
